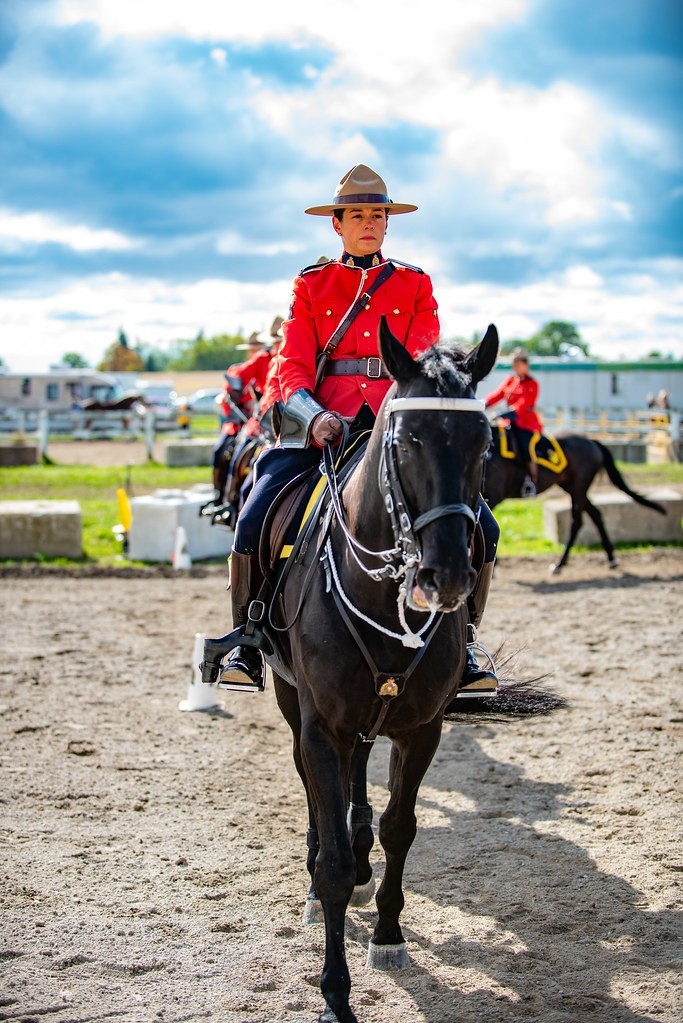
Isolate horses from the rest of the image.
[228,316,501,1022]
[484,421,669,578]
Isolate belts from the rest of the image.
[324,357,391,379]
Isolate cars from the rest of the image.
[179,387,227,415]
[125,381,181,431]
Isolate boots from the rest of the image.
[201,469,226,514]
[216,479,243,525]
[219,551,265,686]
[456,559,499,690]
[515,457,532,494]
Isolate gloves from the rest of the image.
[312,411,344,446]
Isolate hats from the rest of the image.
[235,330,266,350]
[511,347,527,361]
[256,317,284,342]
[305,164,418,216]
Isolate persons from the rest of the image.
[481,356,540,497]
[207,165,503,694]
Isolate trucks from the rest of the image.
[0,368,137,432]
